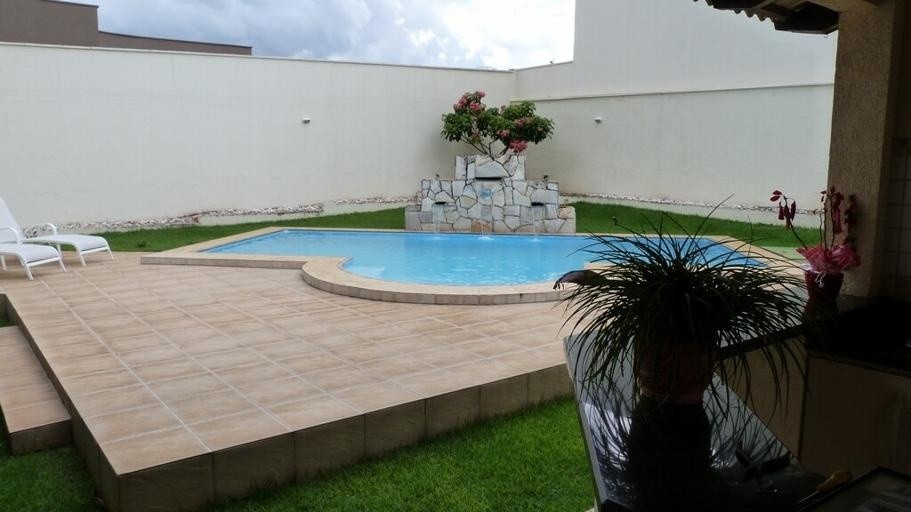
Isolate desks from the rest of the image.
[797,303,911,511]
[561,324,814,512]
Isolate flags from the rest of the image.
[805,272,845,307]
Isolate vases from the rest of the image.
[805,272,845,307]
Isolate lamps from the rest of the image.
[0,196,115,281]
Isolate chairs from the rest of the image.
[0,196,115,281]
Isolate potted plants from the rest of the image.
[553,193,821,430]
[770,184,862,273]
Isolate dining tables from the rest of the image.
[770,184,862,273]
[553,193,821,430]
